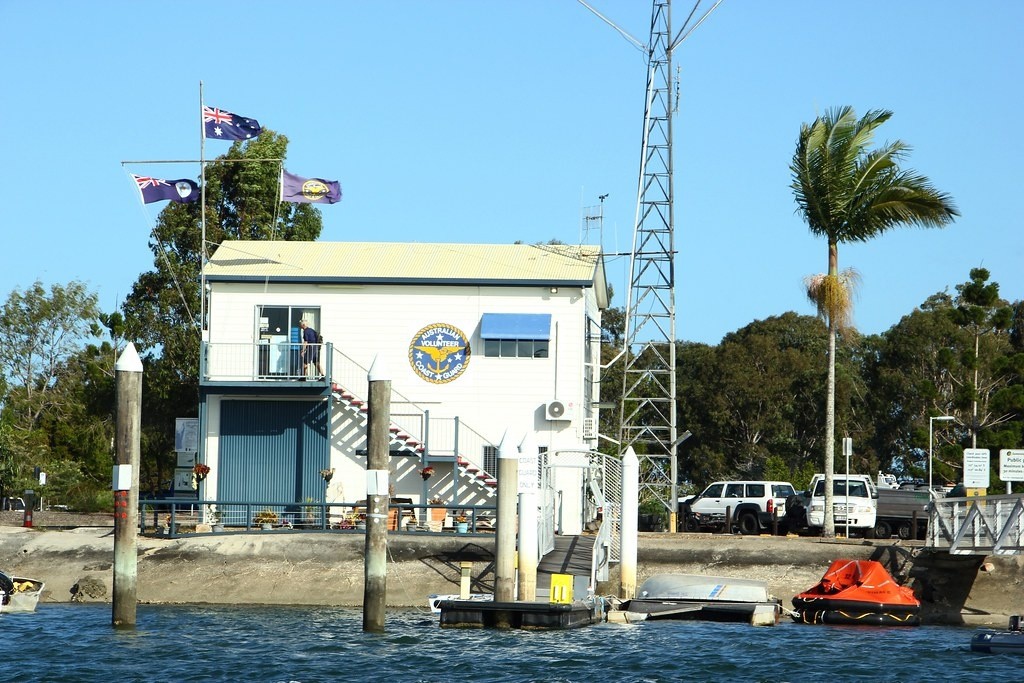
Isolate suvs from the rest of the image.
[35,505,74,512]
[668,480,798,536]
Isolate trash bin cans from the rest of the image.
[637,514,663,531]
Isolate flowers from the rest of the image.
[429,498,446,504]
[409,518,418,523]
[359,514,366,518]
[192,463,210,490]
[418,466,436,476]
[457,517,466,522]
[254,509,278,527]
[162,513,172,528]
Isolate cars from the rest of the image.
[873,474,955,540]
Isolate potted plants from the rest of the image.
[207,505,224,532]
[298,497,319,522]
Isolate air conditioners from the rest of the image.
[584,418,597,440]
[546,399,575,421]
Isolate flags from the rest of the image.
[204,105,265,141]
[280,168,343,205]
[128,172,199,204]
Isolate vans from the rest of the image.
[3,497,26,512]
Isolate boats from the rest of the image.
[607,573,784,626]
[788,558,922,627]
[970,614,1024,656]
[0,571,45,614]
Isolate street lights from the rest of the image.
[929,415,956,501]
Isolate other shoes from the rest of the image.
[318,376,324,381]
[299,377,306,381]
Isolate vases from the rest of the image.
[198,473,205,481]
[406,523,417,531]
[432,508,445,525]
[168,523,183,533]
[456,523,468,533]
[261,523,272,530]
[422,473,431,481]
[157,527,170,534]
[356,521,366,529]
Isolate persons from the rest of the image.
[296,320,327,382]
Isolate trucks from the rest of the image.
[805,472,879,539]
